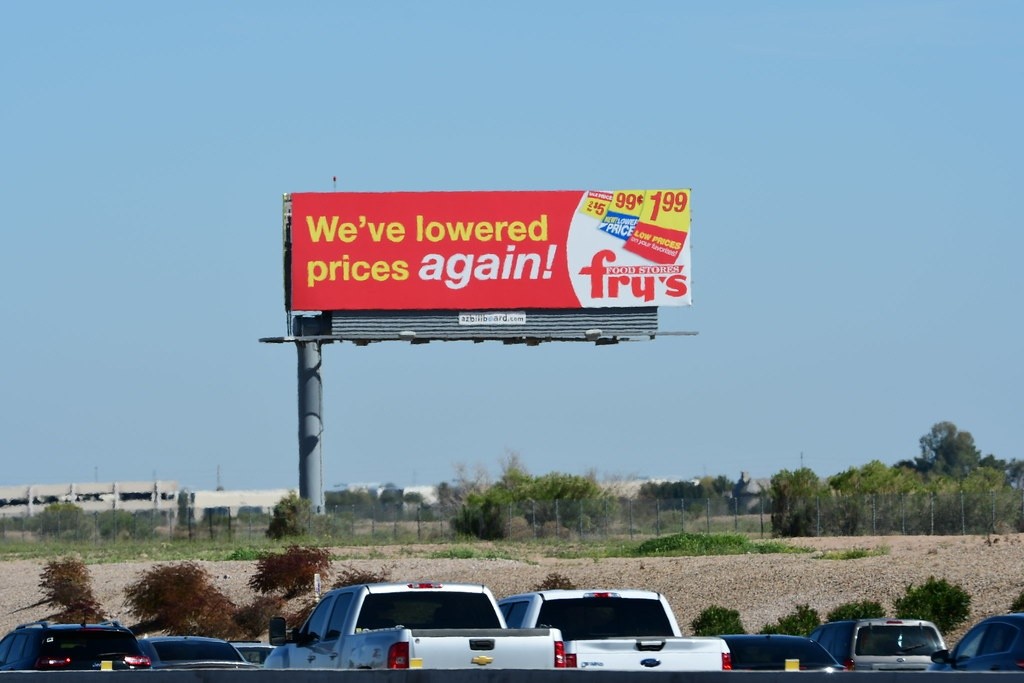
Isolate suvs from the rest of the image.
[0,619,152,671]
[808,619,949,671]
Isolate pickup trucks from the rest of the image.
[263,581,565,672]
[493,588,732,673]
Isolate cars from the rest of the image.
[137,635,261,670]
[716,634,848,672]
[926,612,1024,671]
[226,639,281,670]
